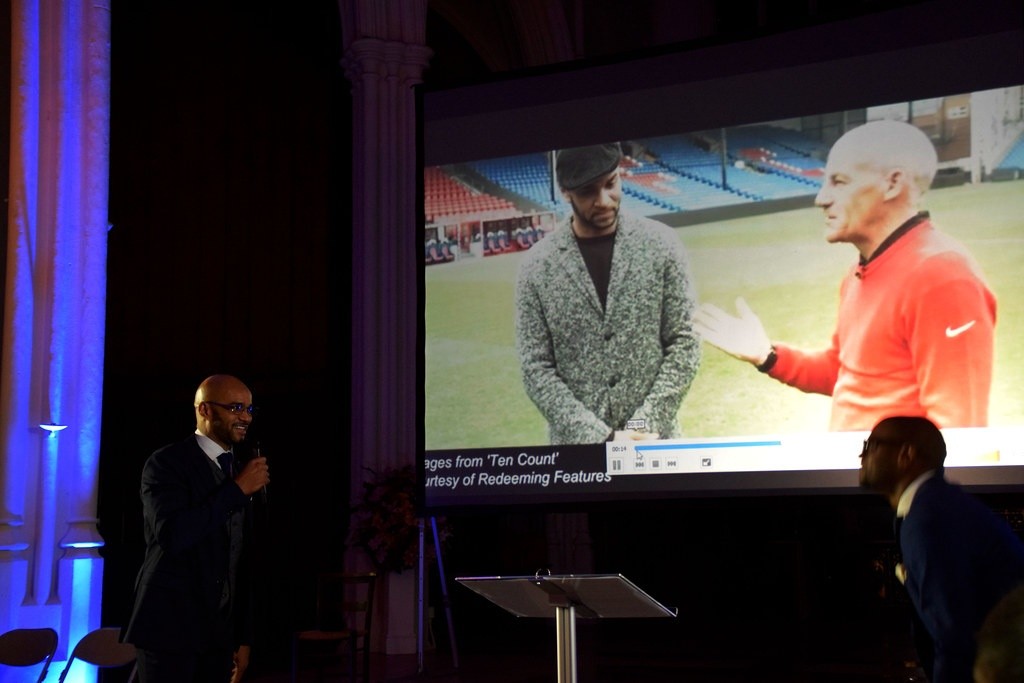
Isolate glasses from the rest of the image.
[862,437,901,453]
[204,401,253,415]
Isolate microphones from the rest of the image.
[248,439,268,504]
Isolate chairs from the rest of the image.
[295,573,376,683]
[424,124,829,264]
[59,627,137,683]
[0,628,58,683]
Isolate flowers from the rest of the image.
[346,464,453,577]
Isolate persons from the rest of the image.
[515,140,704,446]
[118,373,272,683]
[858,416,1024,683]
[692,120,999,433]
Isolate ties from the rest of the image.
[217,452,233,478]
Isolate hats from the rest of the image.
[556,141,621,190]
